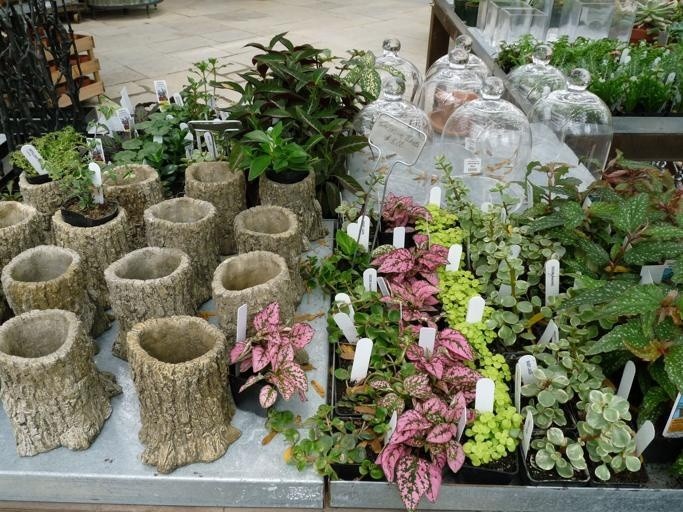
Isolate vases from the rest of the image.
[0,156,330,476]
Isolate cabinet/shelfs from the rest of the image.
[334,110,603,234]
[1,218,339,509]
[419,1,682,164]
[323,337,683,510]
[28,31,106,113]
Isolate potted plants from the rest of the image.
[0,30,409,231]
[328,154,683,509]
[442,1,682,122]
